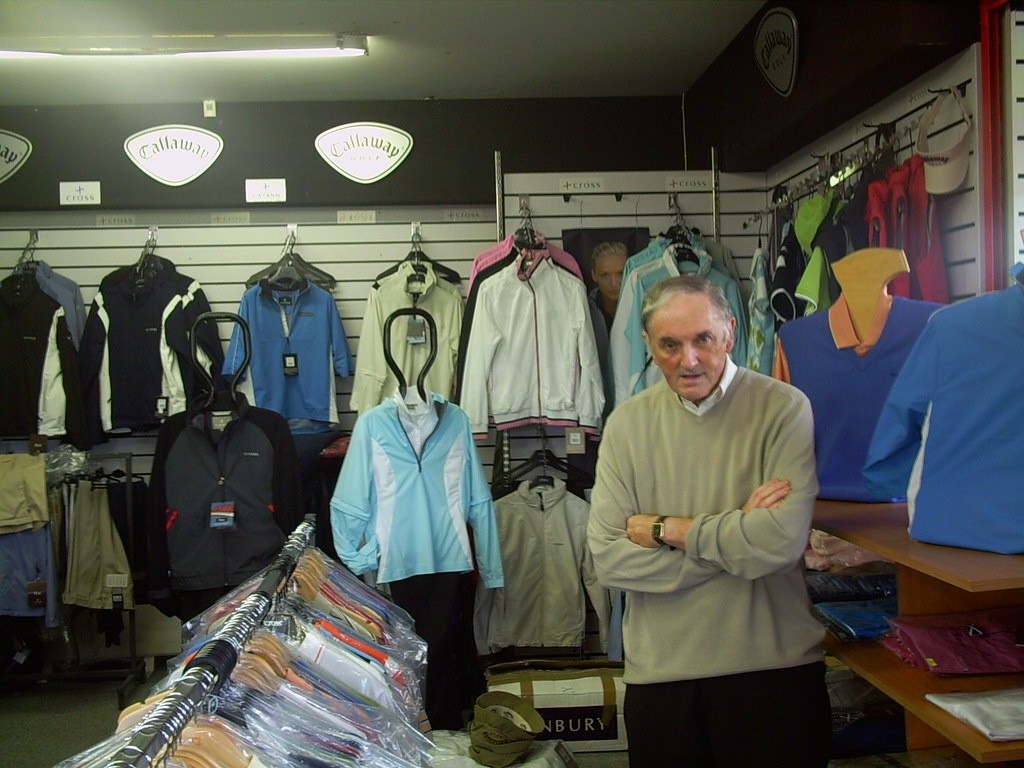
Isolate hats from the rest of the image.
[467,691,545,768]
[914,86,971,194]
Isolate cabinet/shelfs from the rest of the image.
[810,504,1024,767]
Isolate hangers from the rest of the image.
[1,128,938,505]
[56,513,395,768]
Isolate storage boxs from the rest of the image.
[486,660,627,753]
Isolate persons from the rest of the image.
[585,272,830,768]
[589,241,630,332]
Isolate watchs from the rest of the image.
[650,516,677,552]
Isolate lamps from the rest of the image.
[2,30,369,60]
